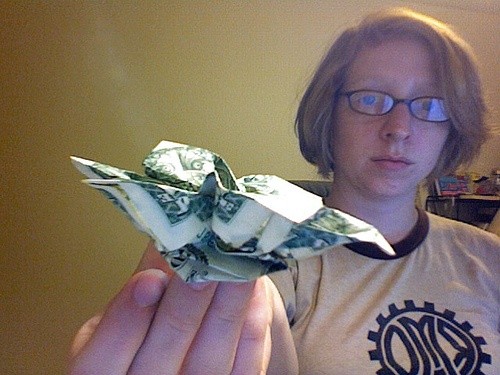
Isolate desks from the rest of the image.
[423,194,500,235]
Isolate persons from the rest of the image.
[68,8,500,375]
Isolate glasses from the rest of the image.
[339,89,453,123]
